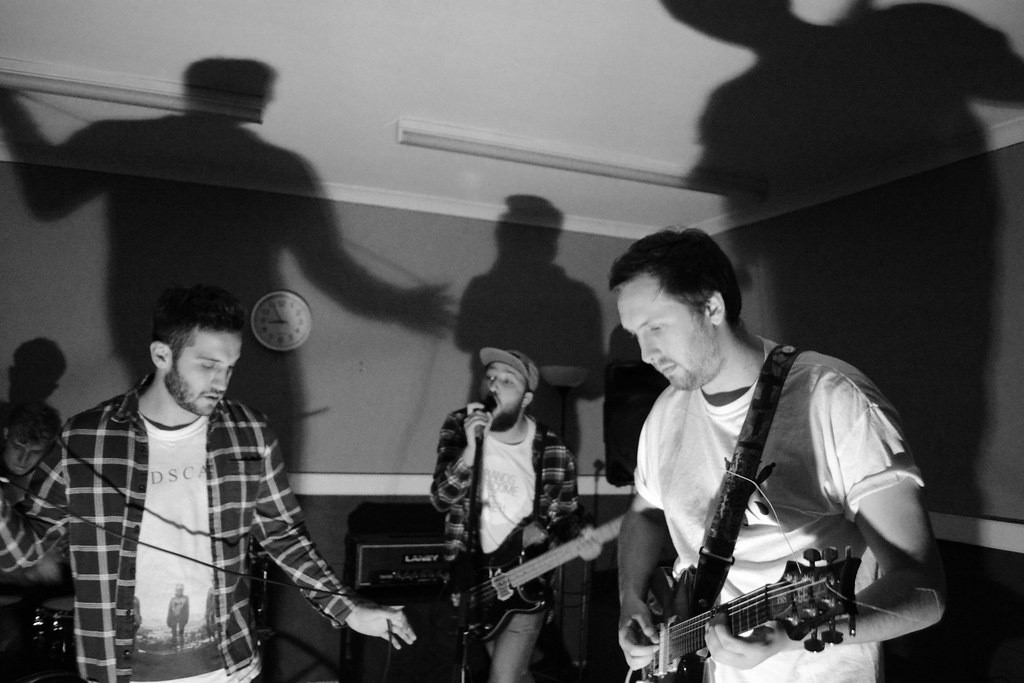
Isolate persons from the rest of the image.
[0,401,72,650]
[132,596,142,650]
[0,281,419,683]
[204,573,217,639]
[429,346,605,683]
[166,580,190,648]
[607,224,949,683]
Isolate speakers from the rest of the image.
[345,532,484,683]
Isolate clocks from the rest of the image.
[250,290,314,352]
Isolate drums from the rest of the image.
[34,597,75,655]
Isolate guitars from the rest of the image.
[629,546,860,683]
[429,514,625,649]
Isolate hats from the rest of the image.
[479,345,541,392]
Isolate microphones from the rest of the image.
[475,396,496,440]
